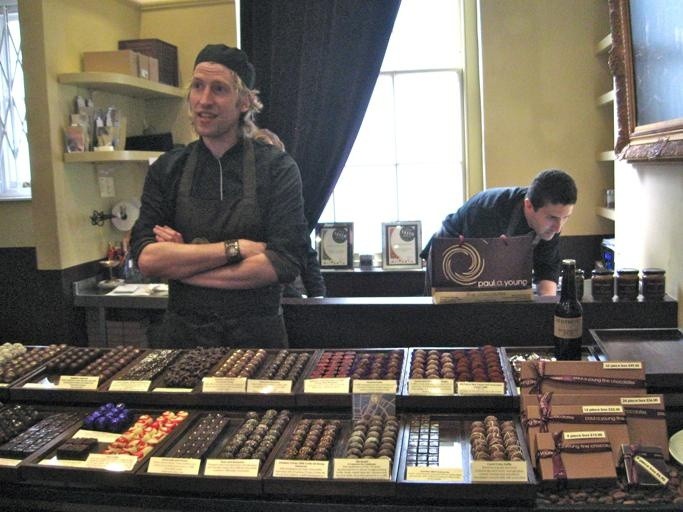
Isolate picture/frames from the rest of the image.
[380,219,422,270]
[606,0,682,162]
[313,221,354,271]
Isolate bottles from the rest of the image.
[607,188,614,209]
[552,257,583,361]
[575,269,667,300]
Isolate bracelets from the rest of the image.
[223,239,242,264]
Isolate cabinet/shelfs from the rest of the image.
[593,27,616,224]
[57,67,185,165]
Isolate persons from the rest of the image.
[283,246,327,298]
[419,169,577,296]
[129,44,311,347]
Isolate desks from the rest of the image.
[1,445,682,510]
[279,287,679,350]
[313,263,427,297]
[72,272,174,350]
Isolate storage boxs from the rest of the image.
[79,50,160,81]
[117,38,180,86]
[123,131,175,152]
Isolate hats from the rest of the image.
[193,43,258,90]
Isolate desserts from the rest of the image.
[0,338,528,466]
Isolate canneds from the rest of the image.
[575,268,665,300]
